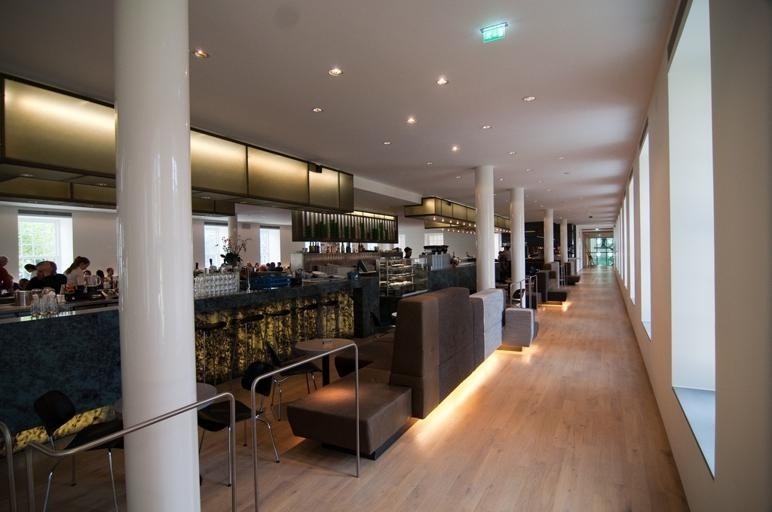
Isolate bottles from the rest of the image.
[195,265,240,300]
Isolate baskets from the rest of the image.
[195,274,239,299]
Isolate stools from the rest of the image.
[525,275,580,338]
[286,335,412,460]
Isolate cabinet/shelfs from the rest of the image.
[379,256,416,328]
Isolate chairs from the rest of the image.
[198,361,279,487]
[266,342,322,421]
[506,289,525,308]
[34,390,124,512]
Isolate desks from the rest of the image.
[295,338,354,387]
[115,383,217,417]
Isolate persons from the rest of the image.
[237,262,318,292]
[404,247,413,265]
[499,244,512,278]
[0,255,120,308]
